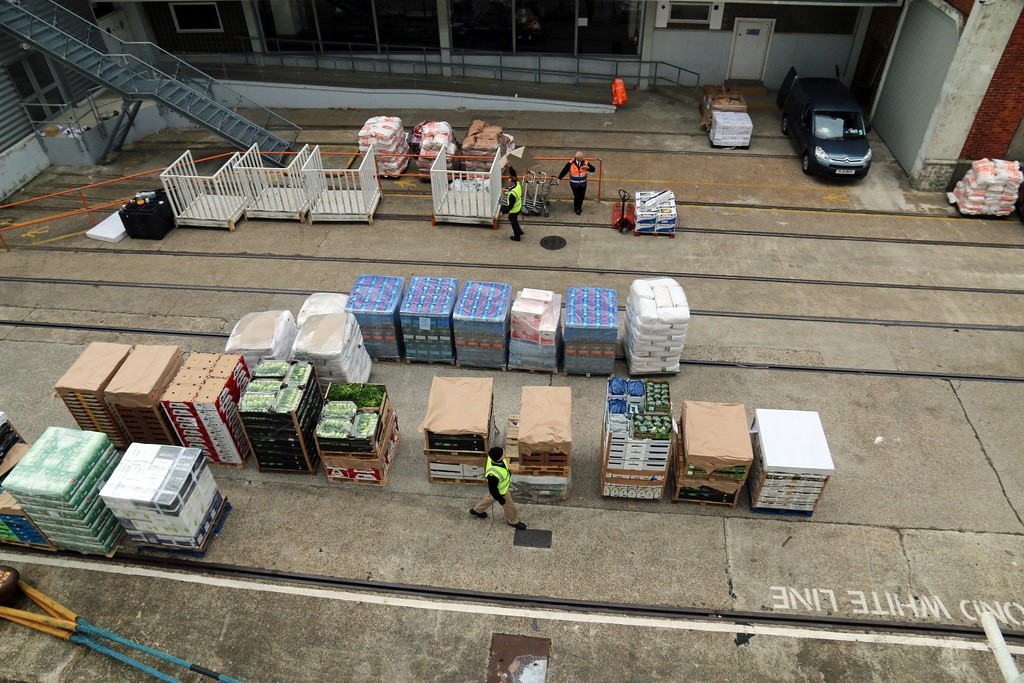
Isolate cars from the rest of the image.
[776,64,873,184]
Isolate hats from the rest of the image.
[488,447,503,461]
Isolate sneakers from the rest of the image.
[518,231,524,236]
[469,508,488,519]
[575,207,582,215]
[507,521,526,531]
[510,236,520,241]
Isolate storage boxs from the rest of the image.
[119,191,180,240]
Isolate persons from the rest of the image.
[498,176,525,242]
[469,447,527,531]
[557,151,595,215]
